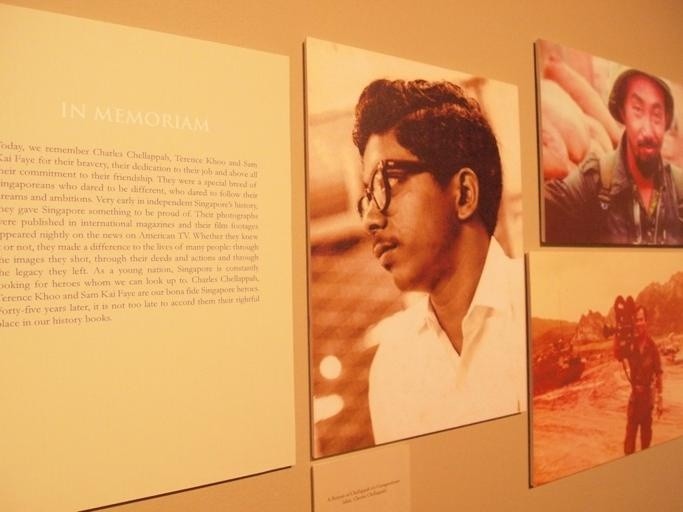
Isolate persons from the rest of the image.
[351,76,526,444]
[543,68,682,244]
[537,44,620,178]
[616,304,666,457]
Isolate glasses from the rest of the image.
[357,159,438,218]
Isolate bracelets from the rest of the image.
[656,392,663,398]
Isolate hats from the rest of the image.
[607,69,676,132]
